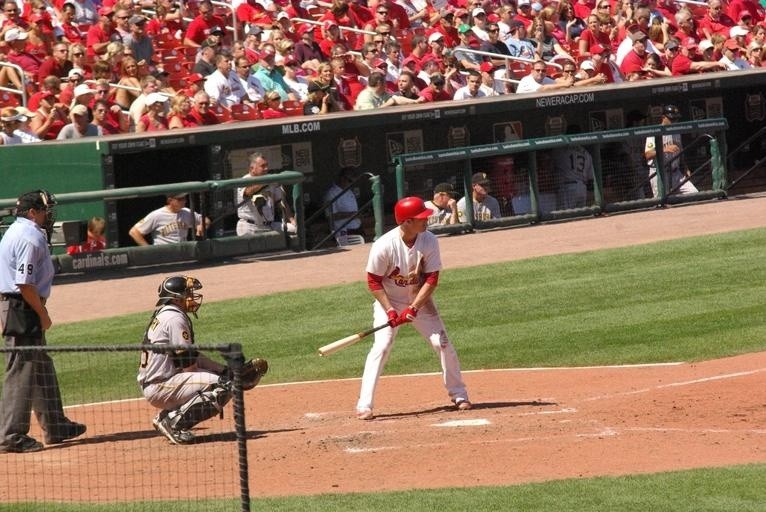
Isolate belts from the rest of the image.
[0,293,46,306]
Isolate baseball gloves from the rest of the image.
[227,357,268,390]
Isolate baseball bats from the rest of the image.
[317,317,396,357]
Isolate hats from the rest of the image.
[433,172,493,196]
[627,104,683,120]
[394,197,434,225]
[166,191,188,199]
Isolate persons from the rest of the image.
[233,150,298,235]
[0,0,765,141]
[490,104,703,216]
[322,166,369,244]
[134,274,268,445]
[0,189,87,454]
[353,196,477,422]
[456,172,502,223]
[127,192,210,247]
[422,182,459,226]
[66,215,106,257]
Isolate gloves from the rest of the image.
[400,305,419,323]
[386,307,399,328]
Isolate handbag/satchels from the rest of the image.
[2,297,42,339]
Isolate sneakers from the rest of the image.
[44,424,86,444]
[457,401,472,410]
[0,442,44,452]
[152,412,196,445]
[355,411,373,420]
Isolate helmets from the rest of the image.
[16,189,58,229]
[156,275,203,319]
